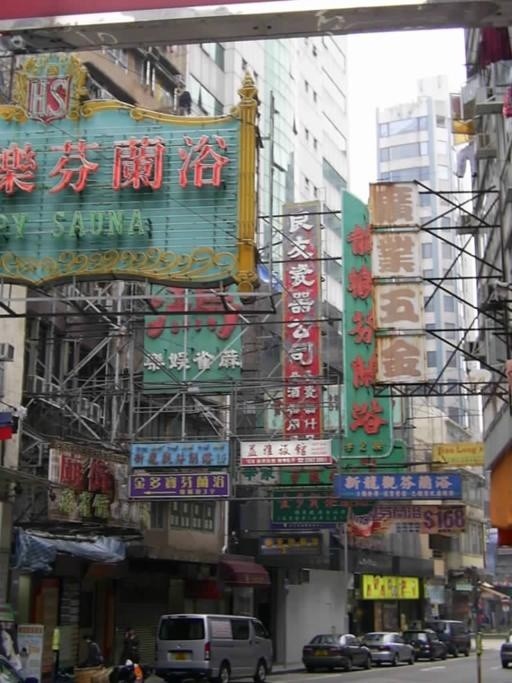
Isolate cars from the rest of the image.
[0,653,24,682]
[497,628,512,668]
[299,616,472,672]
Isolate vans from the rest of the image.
[152,612,274,681]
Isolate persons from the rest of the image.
[0,629,17,659]
[82,627,144,683]
